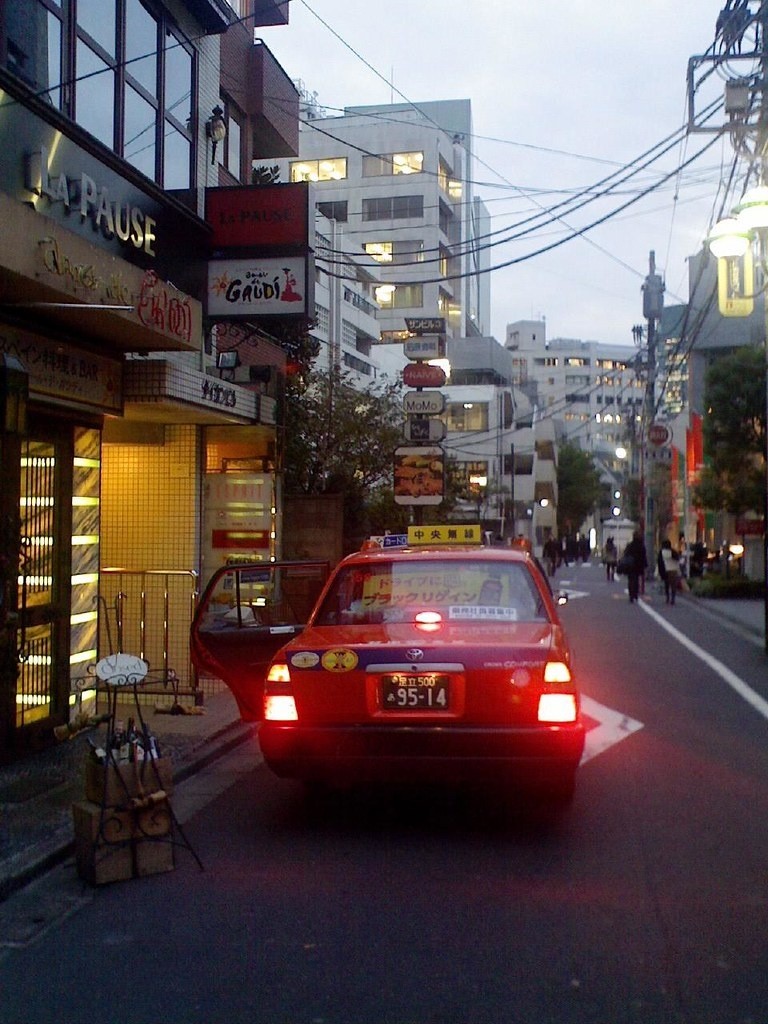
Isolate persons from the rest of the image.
[657,539,682,605]
[616,530,648,603]
[495,531,744,582]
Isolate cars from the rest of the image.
[189,525,585,813]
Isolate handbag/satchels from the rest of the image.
[678,576,690,594]
[617,555,635,575]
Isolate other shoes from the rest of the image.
[630,598,634,603]
[671,601,674,605]
[666,600,669,603]
[634,594,638,599]
